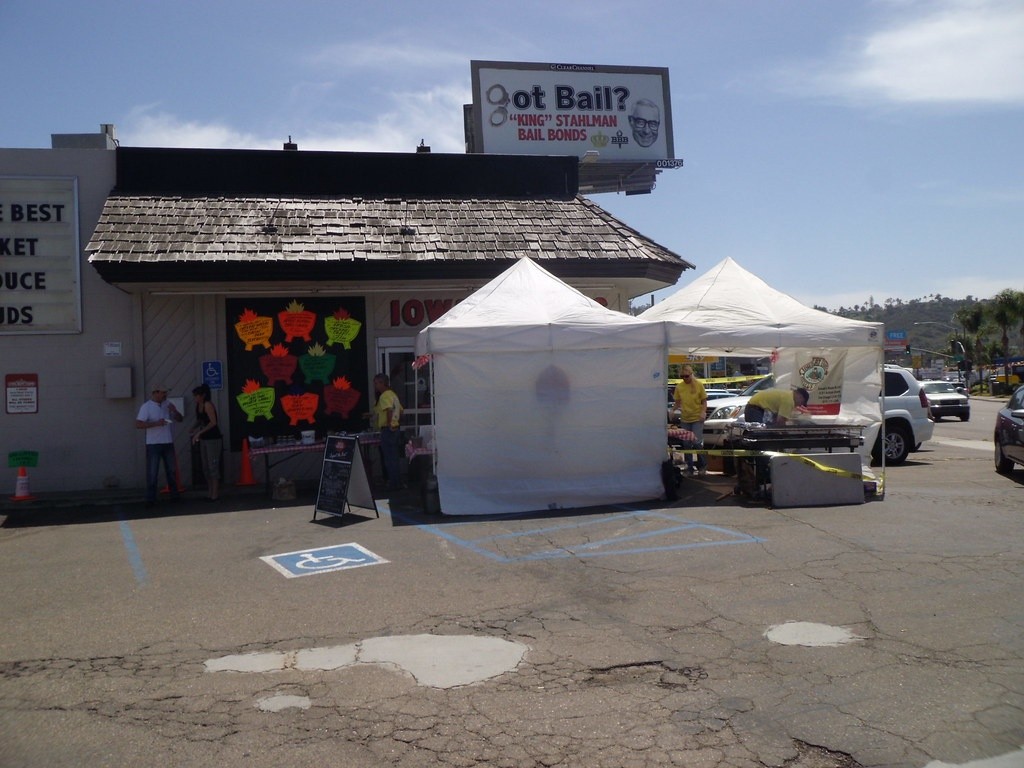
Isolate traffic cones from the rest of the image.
[159,456,185,494]
[9,465,39,503]
[233,438,256,487]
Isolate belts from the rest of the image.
[746,405,764,413]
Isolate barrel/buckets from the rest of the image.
[301,430,315,445]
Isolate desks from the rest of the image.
[249,431,384,494]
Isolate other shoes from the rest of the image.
[682,471,693,478]
[202,496,209,501]
[698,470,706,477]
[206,496,220,502]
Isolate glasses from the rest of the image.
[680,373,692,378]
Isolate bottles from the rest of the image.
[276,435,296,447]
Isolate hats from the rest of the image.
[151,382,171,392]
[797,388,809,407]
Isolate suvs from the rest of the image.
[702,361,934,468]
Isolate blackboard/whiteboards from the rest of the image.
[315,436,356,517]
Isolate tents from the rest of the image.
[415,253,886,515]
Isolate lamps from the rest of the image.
[584,151,600,163]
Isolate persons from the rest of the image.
[628,98,660,147]
[136,385,183,500]
[189,383,224,501]
[374,373,404,502]
[744,388,809,428]
[668,364,708,478]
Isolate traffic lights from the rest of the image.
[906,344,910,354]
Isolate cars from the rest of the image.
[993,385,1024,474]
[704,387,760,400]
[917,379,971,422]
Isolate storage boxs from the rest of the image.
[419,477,441,514]
[272,479,297,500]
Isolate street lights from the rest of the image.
[914,322,961,383]
